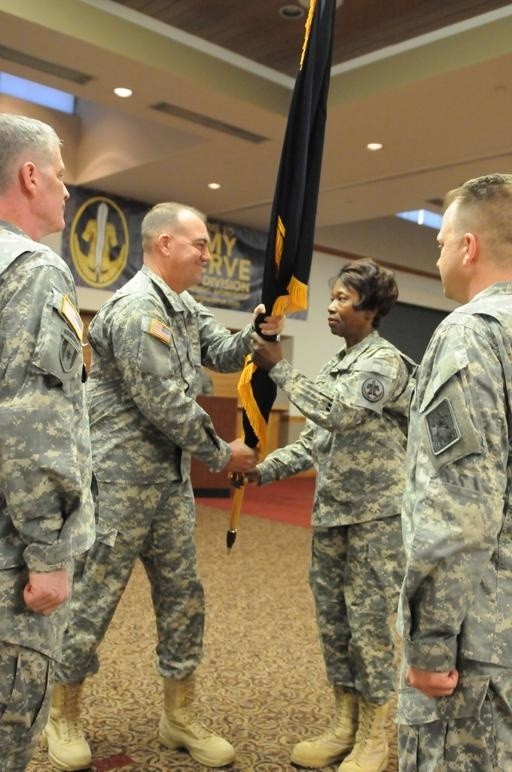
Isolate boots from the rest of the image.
[337,697,389,772]
[43,681,91,771]
[159,673,235,767]
[291,691,358,768]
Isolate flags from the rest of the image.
[232,2,337,470]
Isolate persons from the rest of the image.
[231,257,423,772]
[43,199,285,772]
[0,111,102,772]
[393,171,512,772]
